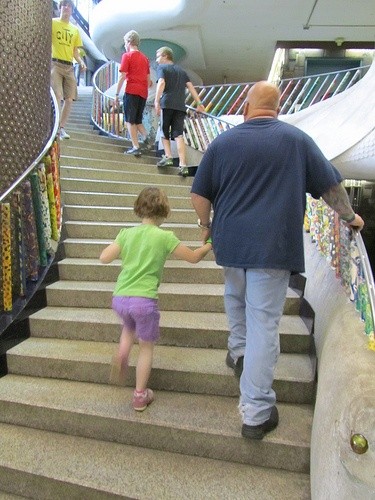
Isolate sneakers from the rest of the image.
[176,166,189,177]
[123,147,142,156]
[241,405,280,440]
[157,154,174,167]
[133,387,154,411]
[111,360,129,387]
[60,127,71,139]
[226,351,245,377]
[144,138,153,150]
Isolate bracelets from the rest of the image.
[205,238,213,245]
[343,212,356,223]
[196,101,204,105]
[116,93,119,97]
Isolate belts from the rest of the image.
[52,58,73,66]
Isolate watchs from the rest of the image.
[197,217,213,231]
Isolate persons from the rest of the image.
[189,81,364,441]
[114,30,154,156]
[152,46,207,176]
[49,0,88,141]
[99,188,213,413]
